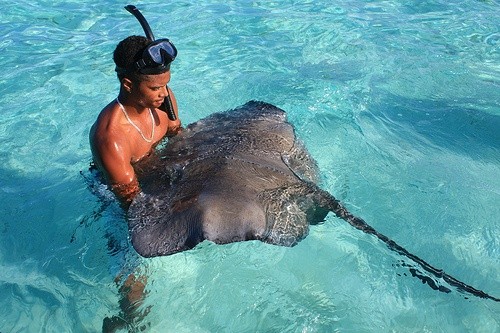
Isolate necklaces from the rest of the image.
[116,97,154,143]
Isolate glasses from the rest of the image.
[133,38,178,72]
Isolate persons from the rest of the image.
[89,35,182,209]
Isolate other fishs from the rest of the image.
[127,100,499,301]
